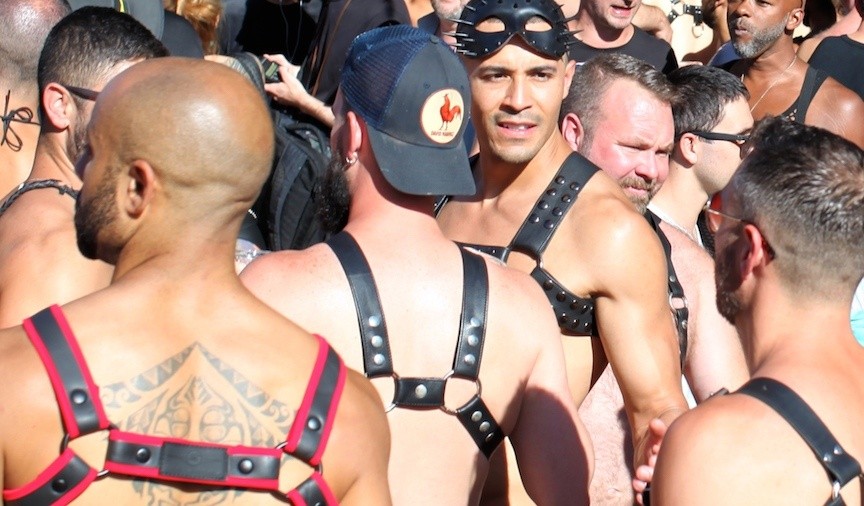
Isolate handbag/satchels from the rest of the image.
[256,110,331,252]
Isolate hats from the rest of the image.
[340,25,476,196]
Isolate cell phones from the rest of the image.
[257,56,283,84]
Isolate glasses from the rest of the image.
[702,191,775,261]
[674,130,753,159]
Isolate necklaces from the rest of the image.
[741,50,799,114]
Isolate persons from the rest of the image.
[236,24,597,506]
[0,56,393,506]
[644,115,864,506]
[0,1,863,505]
[435,0,689,505]
